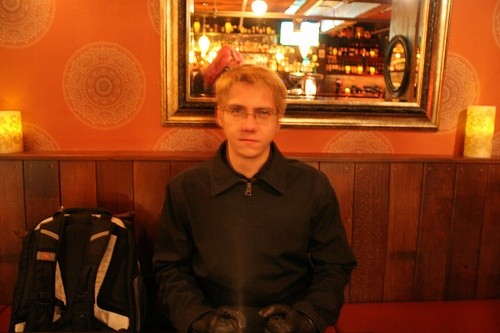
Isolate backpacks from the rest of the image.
[8,208,138,333]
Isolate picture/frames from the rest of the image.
[158,0,450,130]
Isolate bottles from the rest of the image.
[189,18,388,72]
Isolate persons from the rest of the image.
[152,64,358,333]
[193,45,244,95]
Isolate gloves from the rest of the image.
[194,305,260,333]
[258,305,317,333]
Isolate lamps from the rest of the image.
[0,111,24,154]
[463,105,497,159]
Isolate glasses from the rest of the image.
[220,104,278,123]
